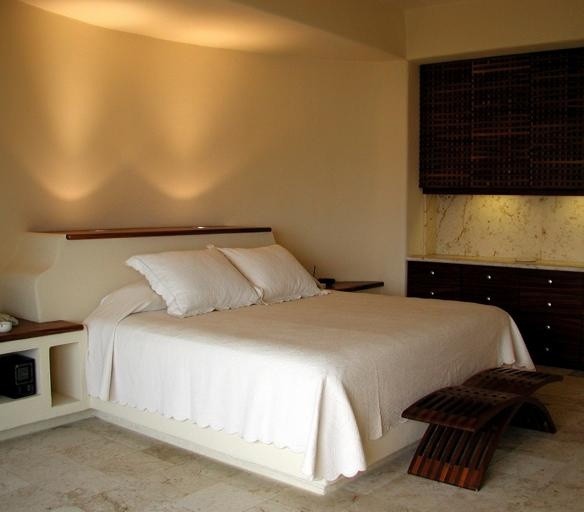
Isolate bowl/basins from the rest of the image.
[318,278,335,287]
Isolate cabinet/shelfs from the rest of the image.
[405,252,583,371]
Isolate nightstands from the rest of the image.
[0,314,91,444]
[325,278,385,295]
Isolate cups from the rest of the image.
[0,322,12,332]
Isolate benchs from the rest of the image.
[399,364,564,493]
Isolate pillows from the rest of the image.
[123,242,266,321]
[205,243,334,306]
[86,282,171,403]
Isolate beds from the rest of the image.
[0,227,540,500]
